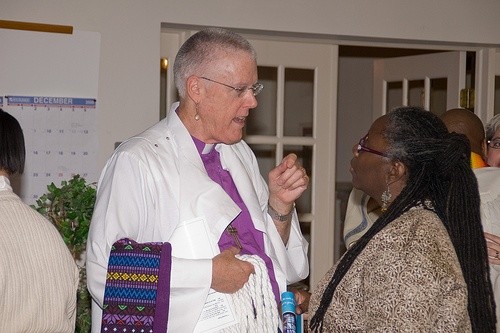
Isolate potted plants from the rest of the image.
[29,174,98,333]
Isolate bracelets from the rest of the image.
[267,198,297,221]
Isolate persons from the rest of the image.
[86,28,309,333]
[307,105,498,333]
[0,108,79,333]
[437,107,500,333]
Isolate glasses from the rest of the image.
[357,137,391,160]
[487,139,500,149]
[201,75,263,96]
